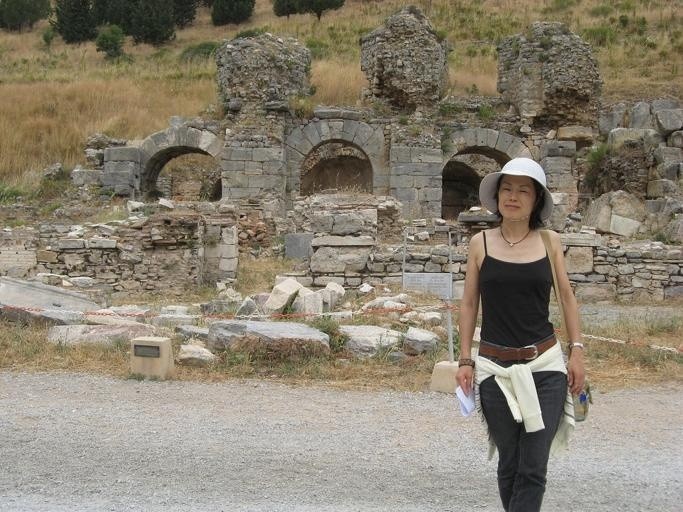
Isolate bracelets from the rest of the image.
[568,342,584,351]
[457,359,475,369]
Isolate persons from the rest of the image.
[454,158,586,512]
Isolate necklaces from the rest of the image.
[500,223,532,247]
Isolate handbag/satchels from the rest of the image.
[573,382,592,421]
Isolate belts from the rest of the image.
[479,336,556,361]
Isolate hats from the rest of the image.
[480,158,554,221]
